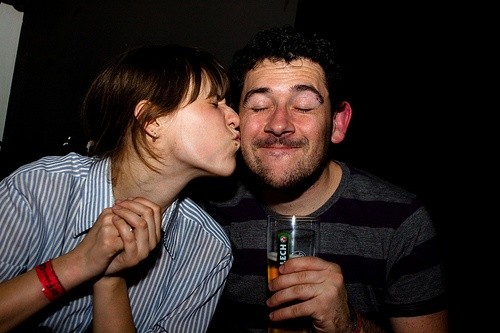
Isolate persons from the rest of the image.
[0,43,242,333]
[178,24,451,333]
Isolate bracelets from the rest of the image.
[34,259,67,304]
[308,300,362,333]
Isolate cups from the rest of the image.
[266,214,319,333]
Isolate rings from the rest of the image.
[129,227,132,232]
